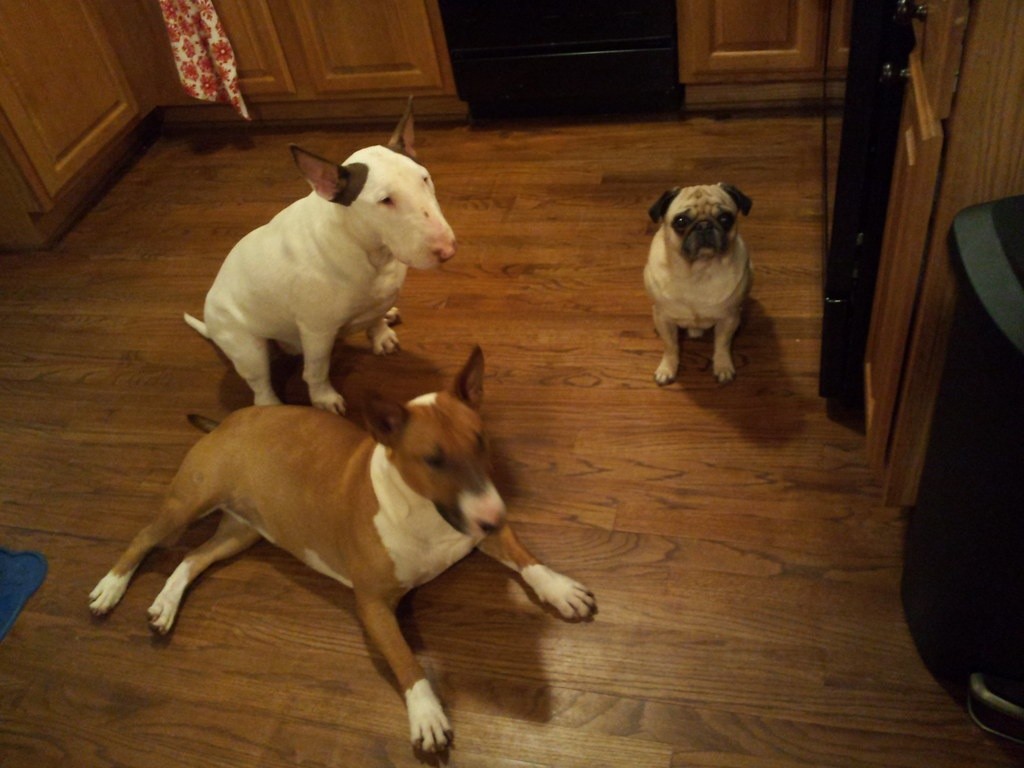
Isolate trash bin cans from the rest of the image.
[897,194,1024,720]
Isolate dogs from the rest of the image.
[183,94,457,416]
[89,346,597,755]
[642,182,752,385]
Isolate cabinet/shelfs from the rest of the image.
[865,0,1024,507]
[677,0,855,114]
[0,0,471,252]
